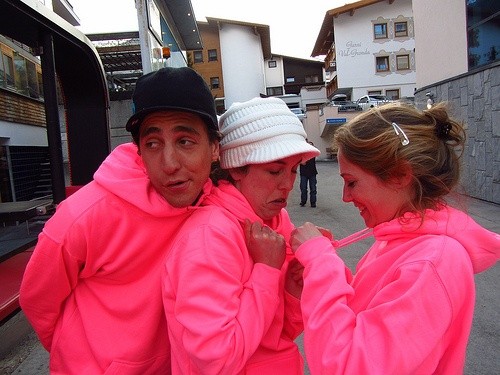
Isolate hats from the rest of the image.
[126,67,219,132]
[217,97,321,169]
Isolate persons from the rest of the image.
[165,94,304,375]
[298,136,318,208]
[289,102,475,375]
[19,68,220,374]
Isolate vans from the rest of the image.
[328,93,348,111]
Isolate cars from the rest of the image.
[356,93,391,112]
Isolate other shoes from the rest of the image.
[311,204,317,208]
[300,202,306,206]
